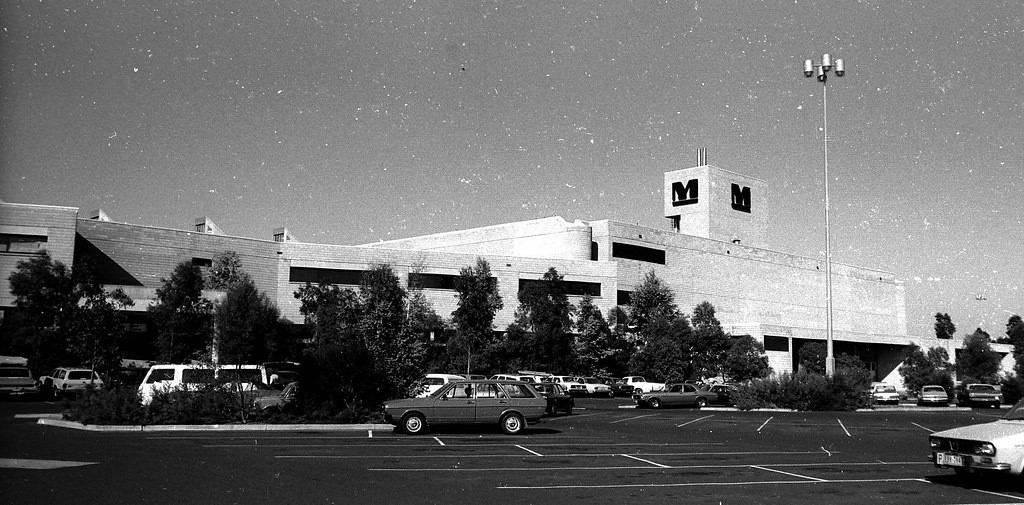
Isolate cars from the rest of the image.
[957,383,1003,409]
[927,396,1024,488]
[39,367,104,401]
[196,362,303,415]
[380,380,547,435]
[916,385,948,406]
[411,370,635,416]
[700,384,740,408]
[0,356,39,400]
[871,383,900,405]
[632,383,718,409]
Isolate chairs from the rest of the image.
[465,387,472,398]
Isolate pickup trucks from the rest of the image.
[620,376,666,394]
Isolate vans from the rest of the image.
[136,363,268,412]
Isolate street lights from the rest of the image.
[803,53,847,377]
